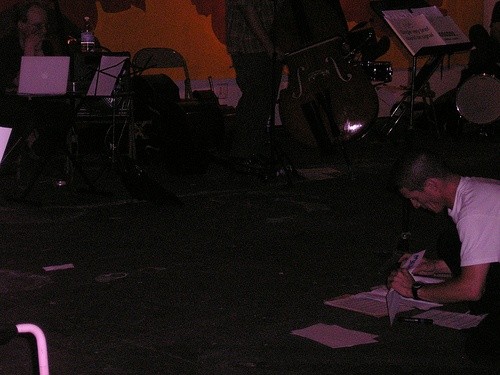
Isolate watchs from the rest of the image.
[412,281,424,299]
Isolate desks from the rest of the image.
[0,91,114,193]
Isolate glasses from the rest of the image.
[28,23,50,30]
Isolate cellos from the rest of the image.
[269,0,380,182]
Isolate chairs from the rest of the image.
[133,46,209,175]
[380,55,444,136]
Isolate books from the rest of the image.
[382,5,470,55]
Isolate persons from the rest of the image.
[0,4,53,87]
[387,149,500,304]
[226,0,281,145]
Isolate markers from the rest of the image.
[396,317,434,326]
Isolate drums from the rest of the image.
[455,72,500,127]
